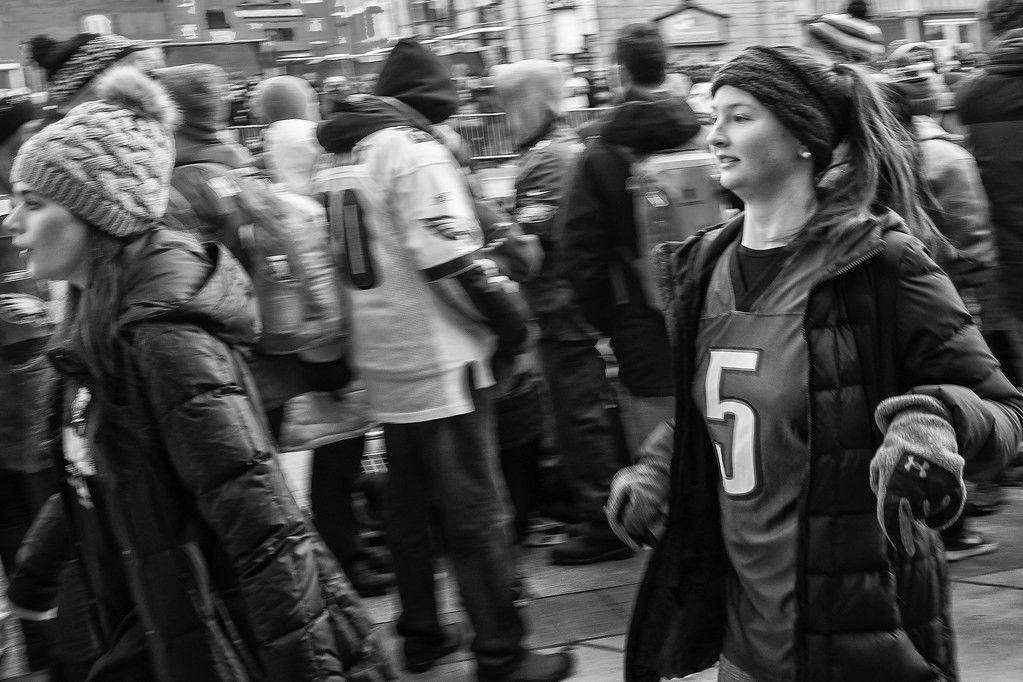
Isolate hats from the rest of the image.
[806,12,981,112]
[12,69,179,236]
[162,62,230,130]
[30,33,154,104]
[0,89,47,149]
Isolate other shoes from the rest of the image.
[350,566,395,593]
[997,464,1022,485]
[969,480,1004,514]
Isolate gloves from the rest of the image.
[607,462,670,550]
[870,415,968,559]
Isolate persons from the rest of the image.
[2,2,1021,680]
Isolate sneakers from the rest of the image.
[478,647,571,682]
[944,531,1000,562]
[406,624,462,672]
[523,519,639,564]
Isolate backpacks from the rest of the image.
[610,136,725,313]
[180,162,340,355]
